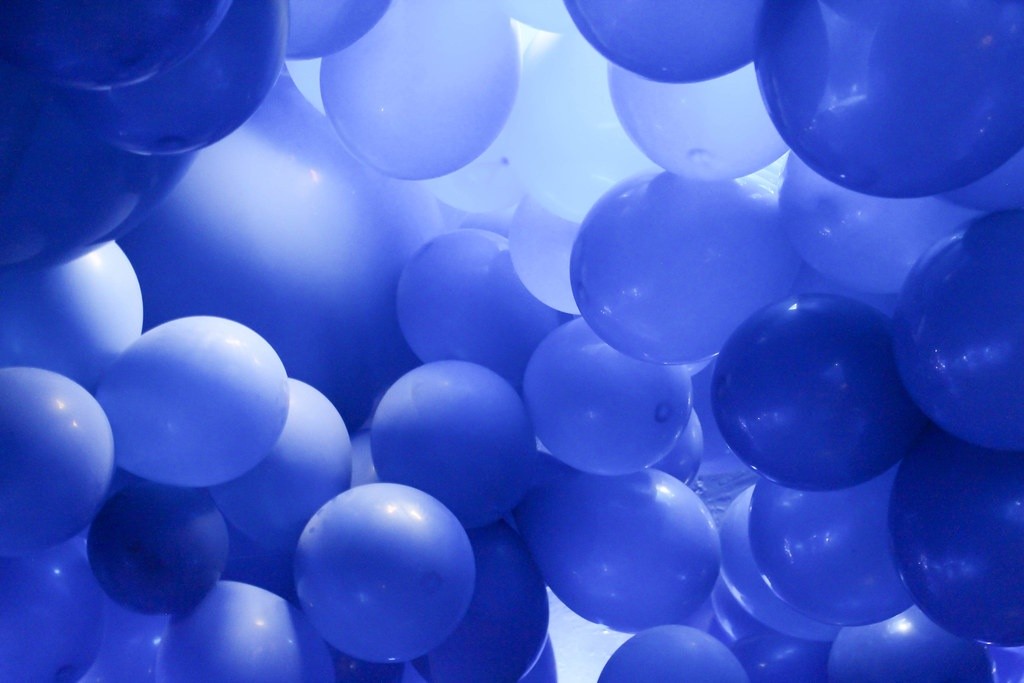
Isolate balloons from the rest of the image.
[0,0,1024,683]
